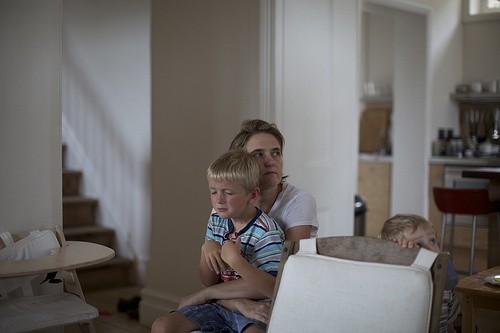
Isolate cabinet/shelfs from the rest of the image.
[358,161,391,238]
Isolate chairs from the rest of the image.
[267,236,452,333]
[0,225,116,333]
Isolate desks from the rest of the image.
[455,264,500,333]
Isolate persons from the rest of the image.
[378,214,458,333]
[190,119,318,333]
[151,148,285,333]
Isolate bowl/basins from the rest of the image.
[457,81,499,93]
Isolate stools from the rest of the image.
[432,186,500,277]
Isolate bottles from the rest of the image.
[450,136,464,158]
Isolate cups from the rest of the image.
[439,128,452,155]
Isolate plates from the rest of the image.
[485,275,500,286]
